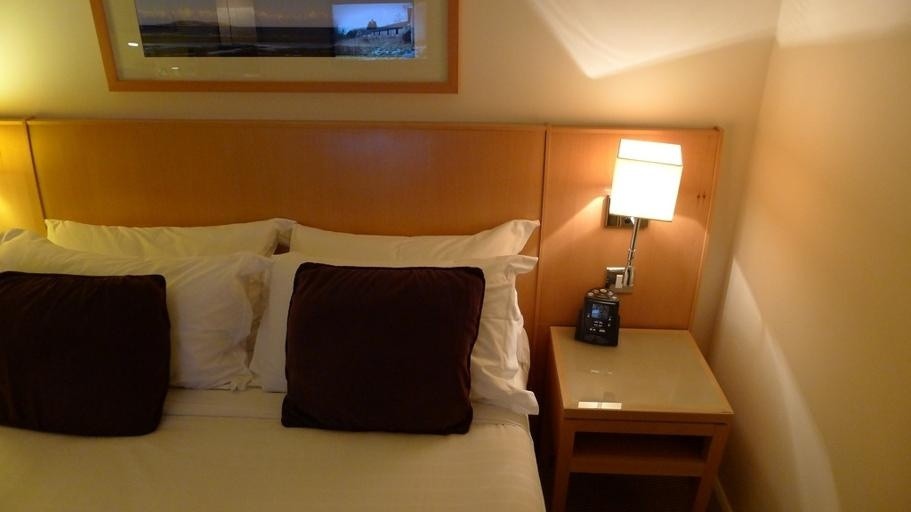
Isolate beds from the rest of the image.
[0,388,548,511]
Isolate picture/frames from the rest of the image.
[90,0,459,93]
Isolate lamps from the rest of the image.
[605,138,685,295]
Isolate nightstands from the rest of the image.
[549,328,734,512]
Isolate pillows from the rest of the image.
[0,271,164,437]
[287,219,540,256]
[262,252,540,417]
[280,261,486,435]
[0,228,272,393]
[44,218,296,312]
